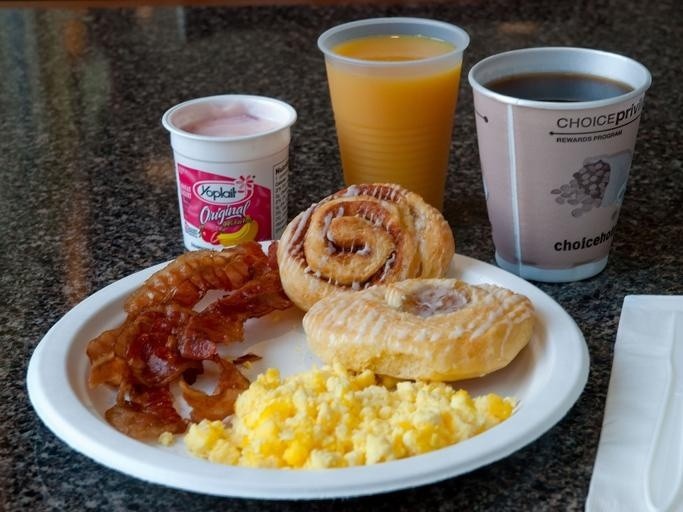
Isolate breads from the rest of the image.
[302,278,534,381]
[274,183,454,316]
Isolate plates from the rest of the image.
[25,239,591,503]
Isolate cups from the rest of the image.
[161,95,298,251]
[470,44,653,285]
[318,17,470,217]
